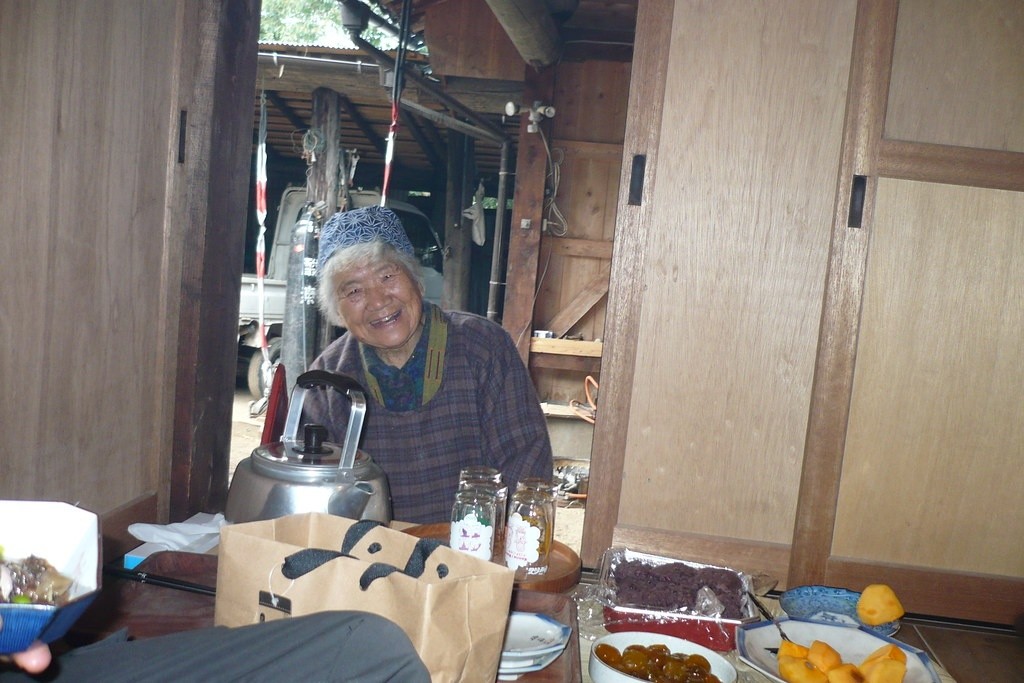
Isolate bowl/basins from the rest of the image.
[588,631,739,683]
[0,499,110,655]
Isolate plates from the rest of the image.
[595,546,760,629]
[495,610,574,680]
[779,584,902,638]
[735,615,943,683]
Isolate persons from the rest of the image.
[0,610,432,683]
[288,205,554,524]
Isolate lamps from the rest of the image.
[505,100,556,133]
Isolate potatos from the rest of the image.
[777,640,908,683]
[857,583,905,626]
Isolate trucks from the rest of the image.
[236,186,452,403]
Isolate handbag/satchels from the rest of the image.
[214,512,516,683]
[462,182,485,246]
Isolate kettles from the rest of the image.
[224,369,394,527]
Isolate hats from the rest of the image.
[317,205,414,266]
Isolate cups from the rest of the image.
[448,465,557,585]
[534,329,553,338]
